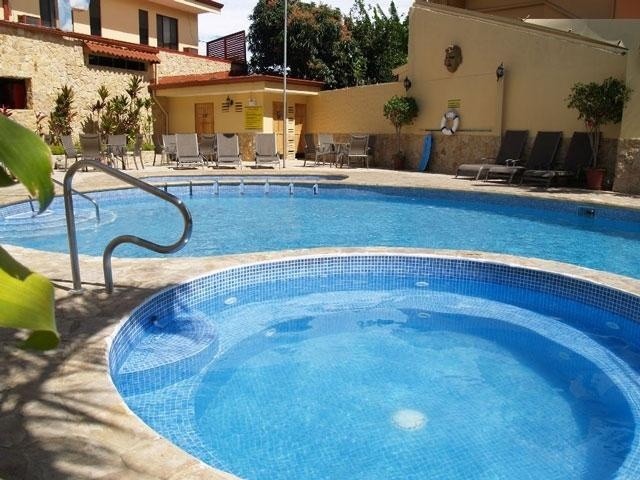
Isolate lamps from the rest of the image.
[404,76,411,92]
[496,61,504,81]
[227,95,233,106]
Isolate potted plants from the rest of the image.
[383,94,419,170]
[563,77,635,190]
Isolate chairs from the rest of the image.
[485,132,562,187]
[60,133,217,172]
[216,131,243,171]
[300,131,370,169]
[455,129,529,181]
[255,131,281,170]
[518,131,603,192]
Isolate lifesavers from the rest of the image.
[440,112,459,136]
